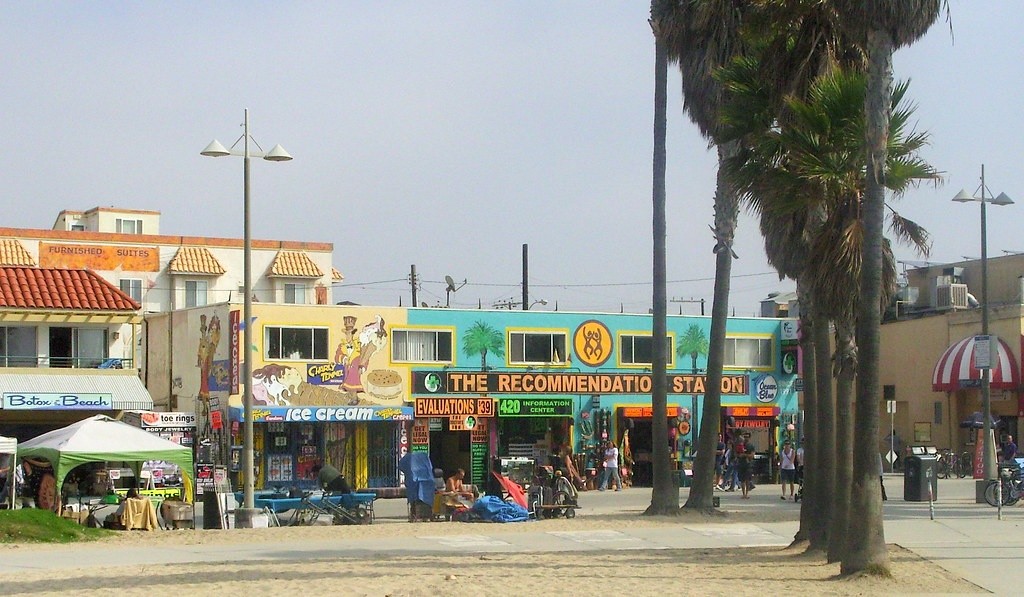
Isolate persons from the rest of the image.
[116,488,137,515]
[445,469,474,502]
[797,438,804,485]
[311,464,351,494]
[777,440,796,500]
[548,447,561,468]
[599,441,622,491]
[563,445,588,491]
[714,430,755,499]
[1003,435,1018,460]
[884,429,901,469]
[880,453,887,501]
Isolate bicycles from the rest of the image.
[983,460,1024,507]
[934,448,974,479]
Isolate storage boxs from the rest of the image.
[63,510,89,527]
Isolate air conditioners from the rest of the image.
[936,284,968,310]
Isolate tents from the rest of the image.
[8,414,195,516]
[0,435,17,510]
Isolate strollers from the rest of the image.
[795,464,803,503]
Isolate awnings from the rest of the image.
[0,374,153,409]
[932,336,1020,393]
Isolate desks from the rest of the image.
[234,491,376,523]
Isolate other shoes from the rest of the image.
[717,485,724,491]
[615,488,622,491]
[748,485,755,490]
[789,496,794,500]
[737,488,742,491]
[599,487,605,491]
[781,496,786,500]
[725,488,734,492]
[742,495,749,499]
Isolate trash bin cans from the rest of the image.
[904,455,937,501]
[201,481,234,529]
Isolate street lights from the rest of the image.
[199,107,294,529]
[950,162,1016,504]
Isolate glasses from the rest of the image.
[785,443,790,445]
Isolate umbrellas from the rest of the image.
[960,411,1005,429]
[492,471,528,509]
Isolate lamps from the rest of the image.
[529,300,547,310]
